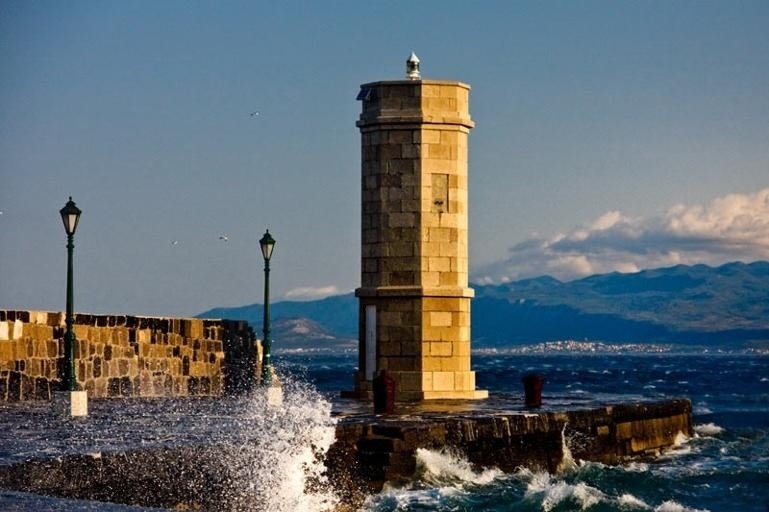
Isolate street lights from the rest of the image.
[258,228,277,387]
[58,194,82,391]
